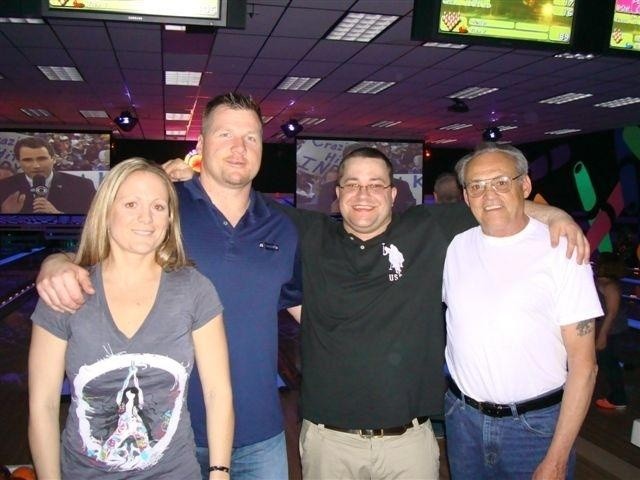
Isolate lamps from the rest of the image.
[280,121,304,137]
[424,149,430,157]
[482,127,502,144]
[114,110,137,131]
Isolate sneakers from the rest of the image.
[595,396,629,411]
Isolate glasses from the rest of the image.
[338,181,390,194]
[464,174,525,199]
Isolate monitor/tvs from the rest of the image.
[590,0,640,58]
[35,0,248,34]
[410,1,590,56]
[0,125,120,235]
[291,135,426,215]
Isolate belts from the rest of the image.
[313,415,429,440]
[448,376,564,419]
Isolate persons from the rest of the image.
[161,148,592,480]
[319,144,416,213]
[441,147,606,480]
[594,252,628,410]
[28,157,237,480]
[433,175,464,204]
[0,137,97,214]
[171,93,302,480]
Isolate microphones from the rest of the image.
[33,173,48,199]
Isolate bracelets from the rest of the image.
[208,466,230,473]
